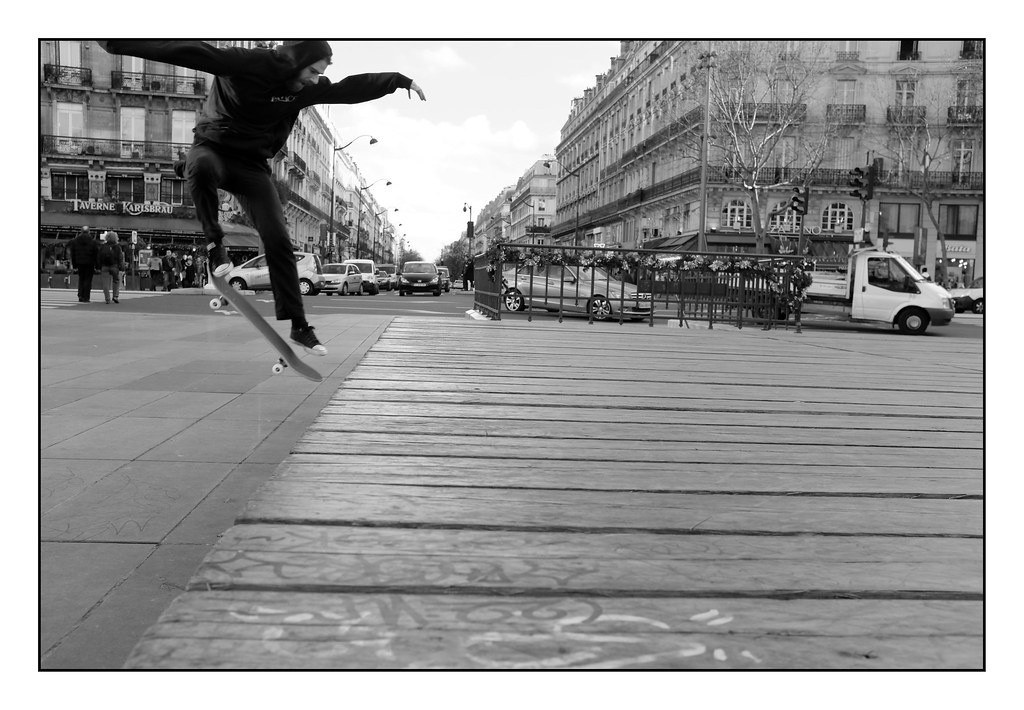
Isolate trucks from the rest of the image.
[718,246,956,337]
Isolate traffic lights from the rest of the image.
[791,187,809,215]
[847,166,873,200]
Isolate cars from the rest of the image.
[373,264,399,292]
[438,266,463,293]
[223,251,328,296]
[322,262,365,296]
[396,260,442,296]
[498,260,652,322]
[945,276,985,316]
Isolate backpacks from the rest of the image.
[101,244,116,267]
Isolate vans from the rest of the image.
[342,259,381,295]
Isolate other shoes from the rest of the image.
[79,297,89,302]
[168,289,171,291]
[161,289,167,291]
[112,297,119,303]
[106,300,110,304]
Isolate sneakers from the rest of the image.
[207,244,234,278]
[290,325,327,356]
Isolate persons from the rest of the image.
[98,234,124,306]
[68,229,100,304]
[133,240,218,292]
[96,39,425,356]
[461,254,477,291]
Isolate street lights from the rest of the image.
[356,178,392,258]
[382,223,402,263]
[372,208,399,260]
[543,159,581,305]
[507,196,535,245]
[327,132,379,262]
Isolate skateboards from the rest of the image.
[203,255,322,382]
